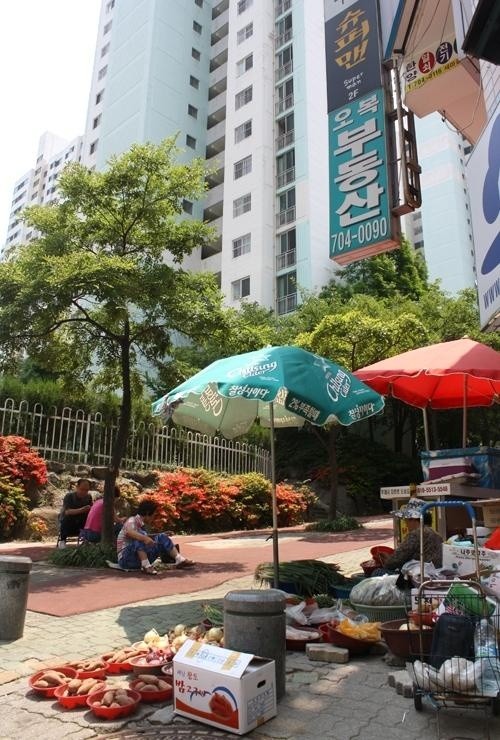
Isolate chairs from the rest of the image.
[56,513,84,545]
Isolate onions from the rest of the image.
[138,624,225,650]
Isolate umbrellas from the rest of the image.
[343,334,500,448]
[151,341,386,589]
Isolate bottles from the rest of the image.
[474,619,499,689]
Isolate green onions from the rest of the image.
[254,558,347,596]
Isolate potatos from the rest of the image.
[32,647,173,709]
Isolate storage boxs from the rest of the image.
[170,639,278,735]
[441,498,500,599]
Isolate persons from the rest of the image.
[78,484,127,542]
[116,498,196,576]
[371,496,444,577]
[56,478,93,548]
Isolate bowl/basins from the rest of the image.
[377,619,437,655]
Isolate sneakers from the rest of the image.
[58,540,67,550]
[176,559,197,570]
[140,566,158,575]
